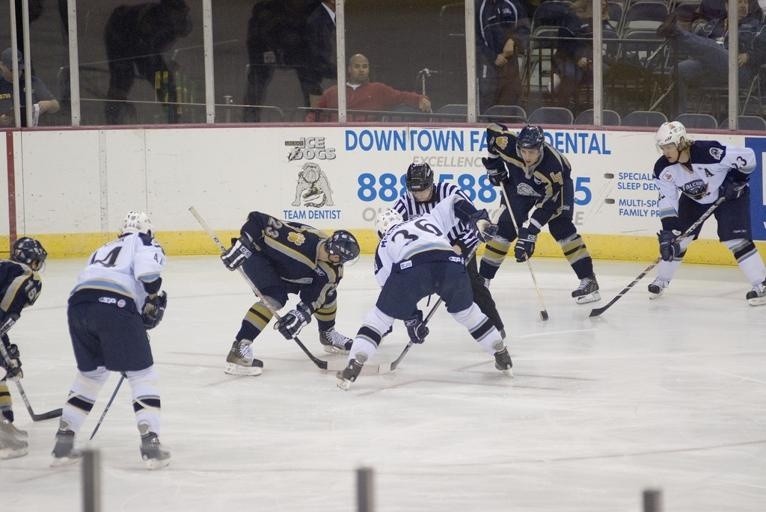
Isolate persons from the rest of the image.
[101,1,190,124]
[395,162,505,344]
[1,1,69,128]
[477,121,601,298]
[305,53,432,124]
[218,211,360,370]
[0,237,47,451]
[242,1,336,123]
[49,209,172,461]
[648,122,766,301]
[477,1,766,119]
[335,193,512,385]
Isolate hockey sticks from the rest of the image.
[190,208,345,369]
[361,205,506,376]
[576,194,726,317]
[1,338,63,421]
[500,181,548,323]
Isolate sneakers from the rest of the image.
[572,273,599,297]
[0,430,28,450]
[657,12,676,35]
[648,276,671,294]
[746,280,766,299]
[336,359,364,382]
[141,432,170,461]
[495,348,513,370]
[226,339,263,368]
[319,326,353,351]
[0,413,27,437]
[51,440,83,459]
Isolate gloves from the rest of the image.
[469,209,498,242]
[514,228,536,262]
[657,230,687,260]
[719,168,751,199]
[221,232,260,271]
[489,172,508,186]
[141,291,167,328]
[0,345,23,379]
[273,305,311,339]
[405,311,429,344]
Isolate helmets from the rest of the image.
[376,209,404,238]
[519,126,544,149]
[655,121,686,147]
[324,230,360,265]
[118,211,154,238]
[10,237,47,270]
[406,163,434,192]
